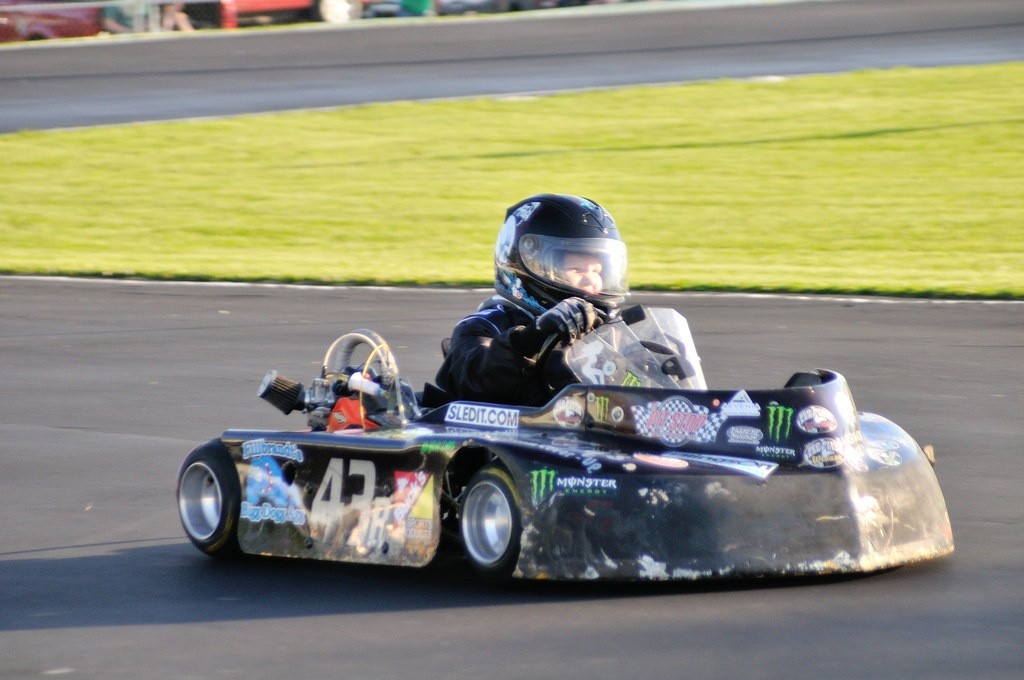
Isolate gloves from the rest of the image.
[533,297,595,347]
[623,340,696,384]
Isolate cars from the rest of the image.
[0,1,103,45]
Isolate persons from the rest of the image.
[101,0,622,38]
[436,193,628,408]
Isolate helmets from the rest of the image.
[494,192,631,328]
